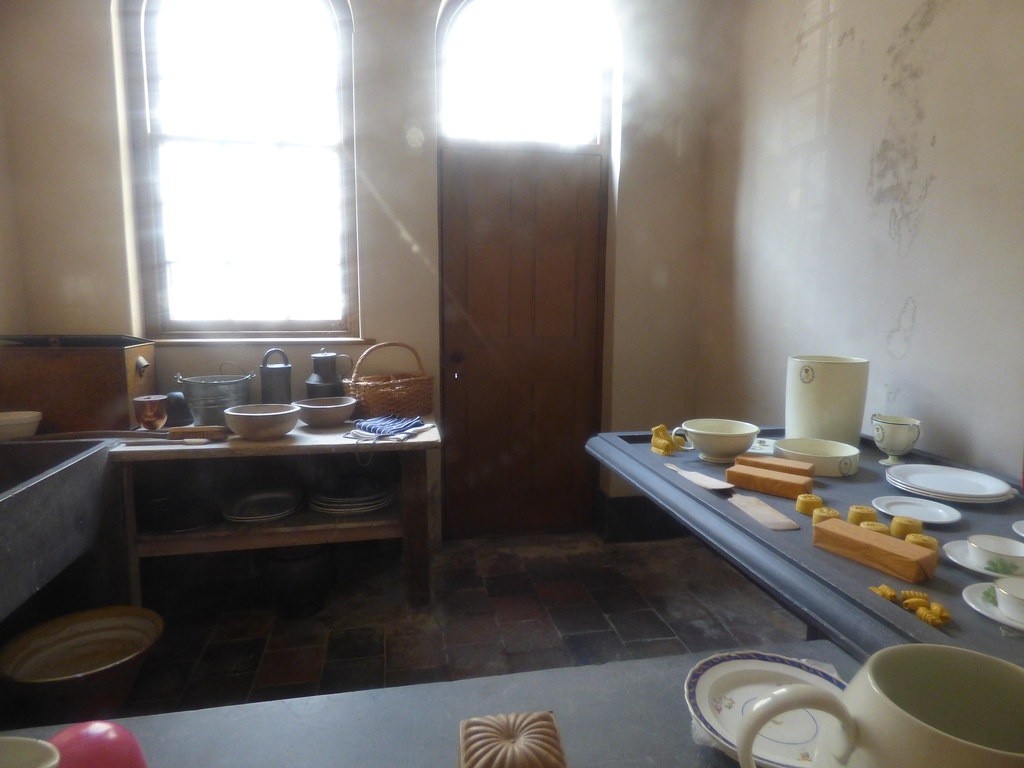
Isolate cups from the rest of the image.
[784,355,869,446]
[872,413,921,466]
[737,644,1024,768]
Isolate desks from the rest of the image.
[0,642,865,768]
[582,423,1024,667]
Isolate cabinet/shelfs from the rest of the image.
[110,411,448,609]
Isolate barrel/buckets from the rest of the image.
[174,372,256,426]
[0,605,164,720]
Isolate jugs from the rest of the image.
[304,348,353,399]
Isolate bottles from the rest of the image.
[260,348,291,404]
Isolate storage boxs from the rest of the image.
[734,452,815,478]
[812,517,937,583]
[0,334,157,434]
[725,464,814,499]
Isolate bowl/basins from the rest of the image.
[224,404,299,441]
[967,535,1024,576]
[292,397,356,426]
[774,437,860,476]
[0,734,62,768]
[995,577,1024,624]
[0,411,42,441]
[671,418,760,463]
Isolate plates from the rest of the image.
[886,463,1018,503]
[140,499,220,532]
[684,652,849,768]
[872,496,961,524]
[222,485,303,522]
[943,540,1024,577]
[309,490,392,514]
[962,583,1024,630]
[1012,520,1024,537]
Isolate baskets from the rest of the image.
[341,341,434,420]
